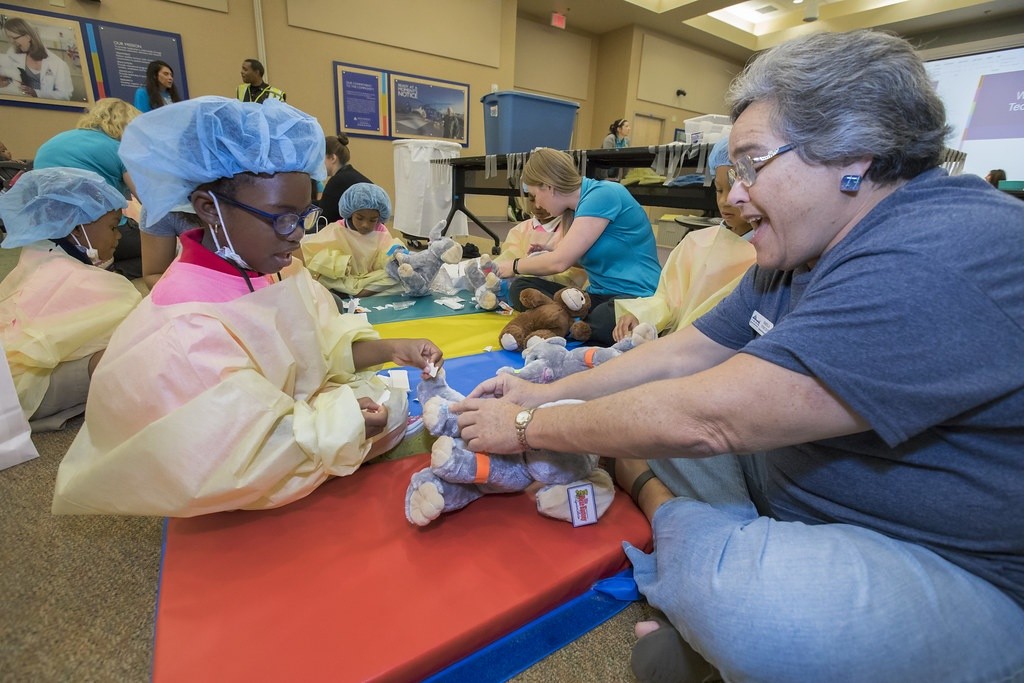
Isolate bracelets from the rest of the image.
[513,258,521,274]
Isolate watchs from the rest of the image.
[515,408,540,451]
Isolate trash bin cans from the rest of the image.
[392,139,462,249]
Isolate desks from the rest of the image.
[427,142,721,256]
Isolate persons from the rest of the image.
[493,193,590,290]
[52,95,444,516]
[497,148,662,347]
[33,97,176,283]
[134,61,182,113]
[595,119,630,180]
[450,29,1024,683]
[0,17,73,101]
[0,167,143,421]
[301,182,392,295]
[313,133,374,224]
[984,169,1007,189]
[236,59,287,104]
[612,137,757,347]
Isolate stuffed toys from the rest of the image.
[499,286,591,351]
[496,323,658,383]
[405,367,615,526]
[464,254,503,311]
[385,220,462,296]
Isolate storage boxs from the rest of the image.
[685,114,731,143]
[481,90,581,155]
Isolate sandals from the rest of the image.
[608,458,656,509]
[629,610,714,682]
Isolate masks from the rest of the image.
[71,225,114,270]
[206,191,254,270]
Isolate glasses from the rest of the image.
[12,35,23,41]
[212,192,323,237]
[727,141,800,187]
